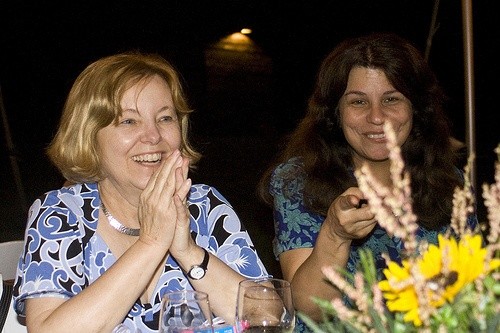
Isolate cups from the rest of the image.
[236,278,295,333]
[159,291,212,333]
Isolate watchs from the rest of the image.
[181,248,209,281]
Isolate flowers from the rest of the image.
[292,119,500,333]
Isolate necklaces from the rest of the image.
[99,195,140,236]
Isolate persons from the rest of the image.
[257,32,487,322]
[12,49,284,333]
